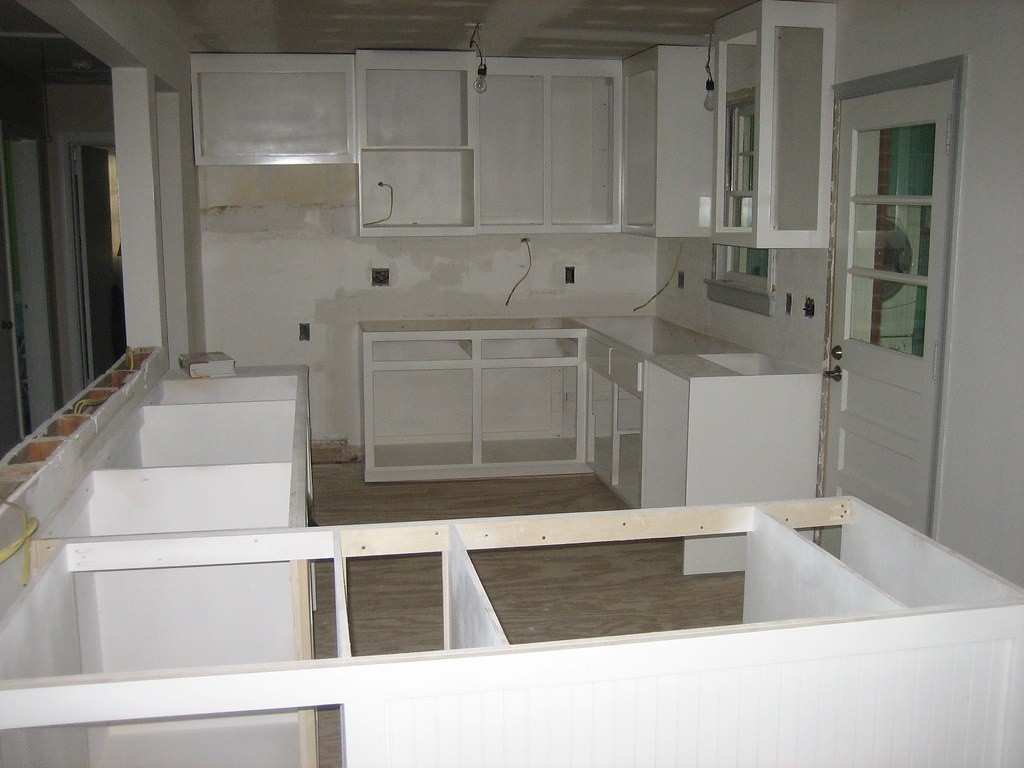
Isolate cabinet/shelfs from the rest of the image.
[562,315,757,509]
[357,51,477,237]
[621,45,713,238]
[189,52,357,166]
[711,0,837,248]
[477,56,622,234]
[641,352,824,573]
[358,317,587,483]
[0,344,319,768]
[305,492,1024,768]
